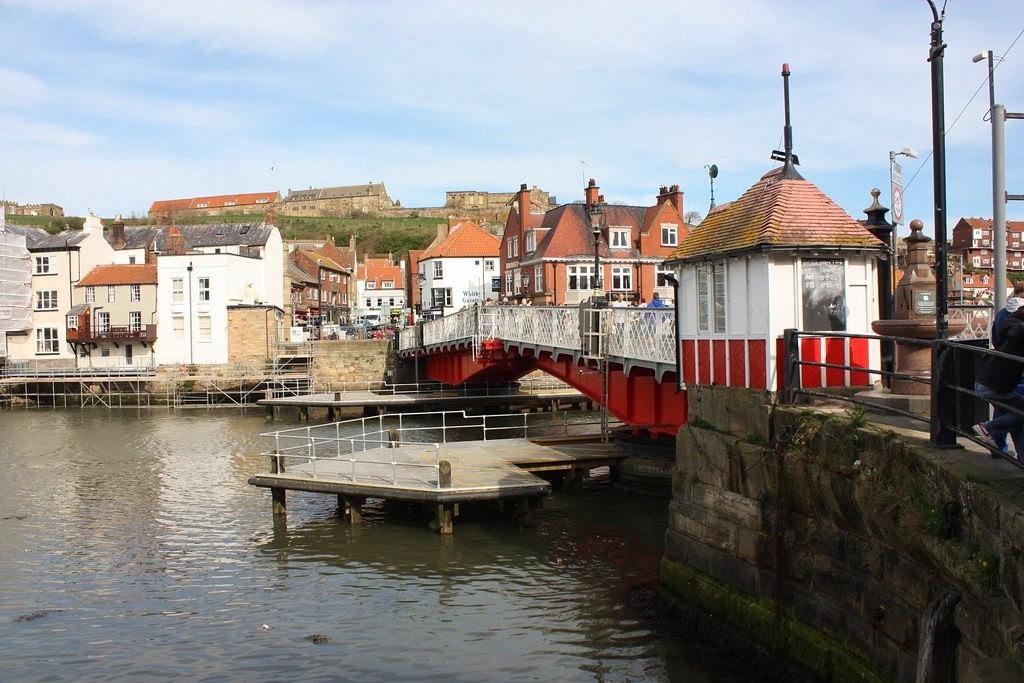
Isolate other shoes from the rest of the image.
[992,450,1016,458]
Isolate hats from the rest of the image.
[1014,282,1024,294]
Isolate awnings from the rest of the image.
[606,291,640,301]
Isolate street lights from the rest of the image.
[585,201,607,301]
[889,148,918,312]
[522,271,530,293]
[417,271,427,320]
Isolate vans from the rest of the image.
[361,311,388,329]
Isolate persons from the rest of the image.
[972,280,1024,468]
[609,292,674,354]
[828,295,846,330]
[459,297,587,341]
[368,330,394,339]
[330,329,337,340]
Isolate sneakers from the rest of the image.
[972,421,997,447]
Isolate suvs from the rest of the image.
[353,320,372,332]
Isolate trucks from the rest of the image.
[388,310,403,328]
